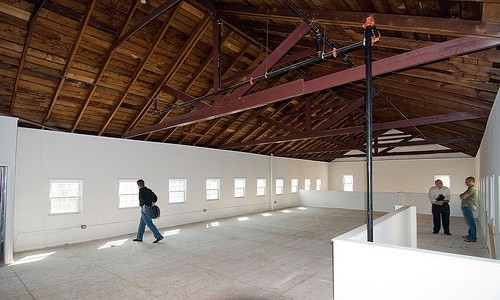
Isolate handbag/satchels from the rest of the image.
[436,194,445,201]
[151,205,160,219]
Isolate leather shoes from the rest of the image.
[444,232,452,235]
[464,239,477,242]
[433,231,439,233]
[462,235,469,238]
[133,239,143,241]
[153,236,163,243]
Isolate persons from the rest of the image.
[428,180,452,235]
[460,177,478,242]
[133,179,164,243]
